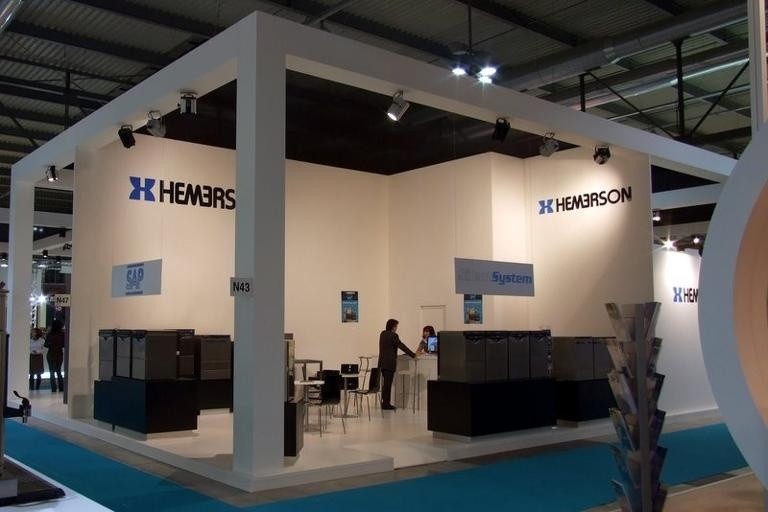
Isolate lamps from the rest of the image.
[45,165,59,183]
[490,116,611,165]
[117,92,197,148]
[448,6,499,84]
[385,92,410,122]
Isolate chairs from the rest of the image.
[293,357,385,438]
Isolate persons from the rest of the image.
[376,318,425,409]
[29,327,43,390]
[416,325,436,354]
[41,320,65,392]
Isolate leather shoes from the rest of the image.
[383,405,396,410]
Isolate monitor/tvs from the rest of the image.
[427,336,438,352]
